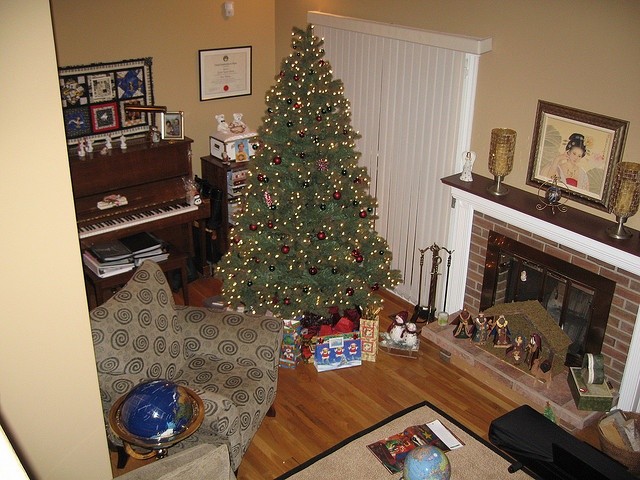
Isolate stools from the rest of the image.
[82,242,190,307]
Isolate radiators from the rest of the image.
[307,10,492,318]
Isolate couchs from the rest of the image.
[90,260,284,479]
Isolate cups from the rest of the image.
[437,311,449,327]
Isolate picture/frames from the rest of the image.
[161,110,184,140]
[58,57,156,147]
[199,46,252,101]
[525,99,630,214]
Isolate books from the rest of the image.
[366,417,466,475]
[82,240,136,279]
[117,231,171,268]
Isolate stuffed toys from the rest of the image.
[402,322,419,348]
[387,310,407,344]
[231,113,250,133]
[214,112,230,134]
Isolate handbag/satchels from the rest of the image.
[195,175,223,230]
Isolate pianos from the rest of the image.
[69,135,211,277]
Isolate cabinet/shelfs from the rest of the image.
[488,248,598,369]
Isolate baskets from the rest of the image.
[598,409,640,477]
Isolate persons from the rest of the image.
[449,308,473,339]
[471,312,486,346]
[522,332,543,368]
[504,332,530,366]
[492,315,510,348]
[540,133,592,192]
[172,119,181,135]
[166,120,173,135]
[236,142,248,161]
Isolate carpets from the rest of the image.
[275,400,543,480]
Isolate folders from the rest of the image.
[82,234,174,279]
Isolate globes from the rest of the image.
[403,445,451,480]
[109,378,205,459]
[534,175,571,216]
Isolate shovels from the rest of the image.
[410,256,424,322]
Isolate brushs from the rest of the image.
[438,257,449,326]
